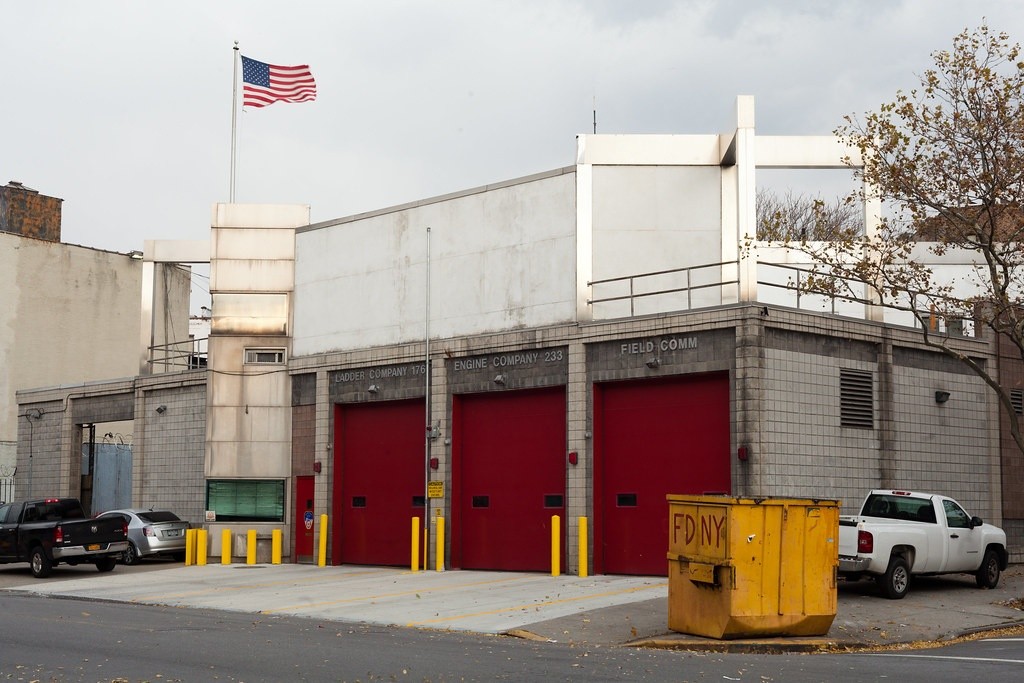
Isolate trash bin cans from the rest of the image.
[667,493,841,641]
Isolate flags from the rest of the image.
[241,55,316,107]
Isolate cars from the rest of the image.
[93,507,191,566]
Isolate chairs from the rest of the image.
[24,507,39,522]
[917,504,935,516]
[878,501,898,514]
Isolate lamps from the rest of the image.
[646,357,659,368]
[155,404,167,414]
[369,385,379,394]
[936,391,950,402]
[494,374,505,386]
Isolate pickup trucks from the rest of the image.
[832,489,1008,600]
[0,496,130,577]
